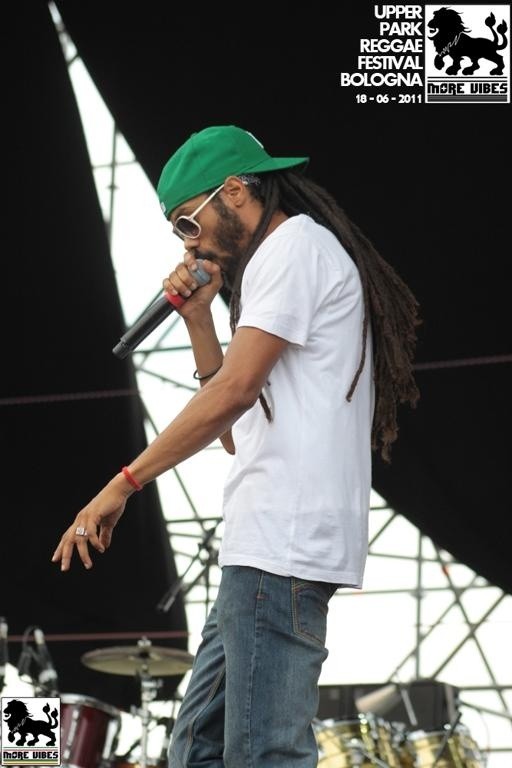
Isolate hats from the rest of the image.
[155,126,312,223]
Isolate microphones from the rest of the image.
[111,258,212,359]
[34,629,59,691]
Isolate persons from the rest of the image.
[50,120,427,767]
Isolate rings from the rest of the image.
[73,526,88,536]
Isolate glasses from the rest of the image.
[171,178,247,241]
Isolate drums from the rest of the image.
[313,716,421,768]
[406,722,487,767]
[59,693,120,768]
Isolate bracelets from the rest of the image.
[121,465,143,492]
[189,360,223,380]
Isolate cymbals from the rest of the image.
[82,646,196,677]
